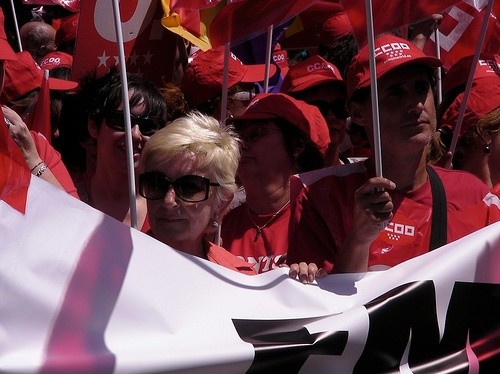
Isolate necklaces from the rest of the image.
[247,199,290,240]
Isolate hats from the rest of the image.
[437,54,500,130]
[281,55,343,94]
[225,93,331,154]
[271,42,290,77]
[39,51,74,70]
[3,50,79,98]
[0,7,18,62]
[346,34,444,96]
[180,46,277,102]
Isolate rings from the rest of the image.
[5,119,11,126]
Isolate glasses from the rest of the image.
[243,125,282,145]
[105,110,157,137]
[138,172,218,202]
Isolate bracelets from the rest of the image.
[36,164,48,177]
[31,161,44,173]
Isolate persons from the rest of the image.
[225,93,330,284]
[60,74,167,236]
[138,111,258,275]
[0,0,500,226]
[286,33,489,275]
